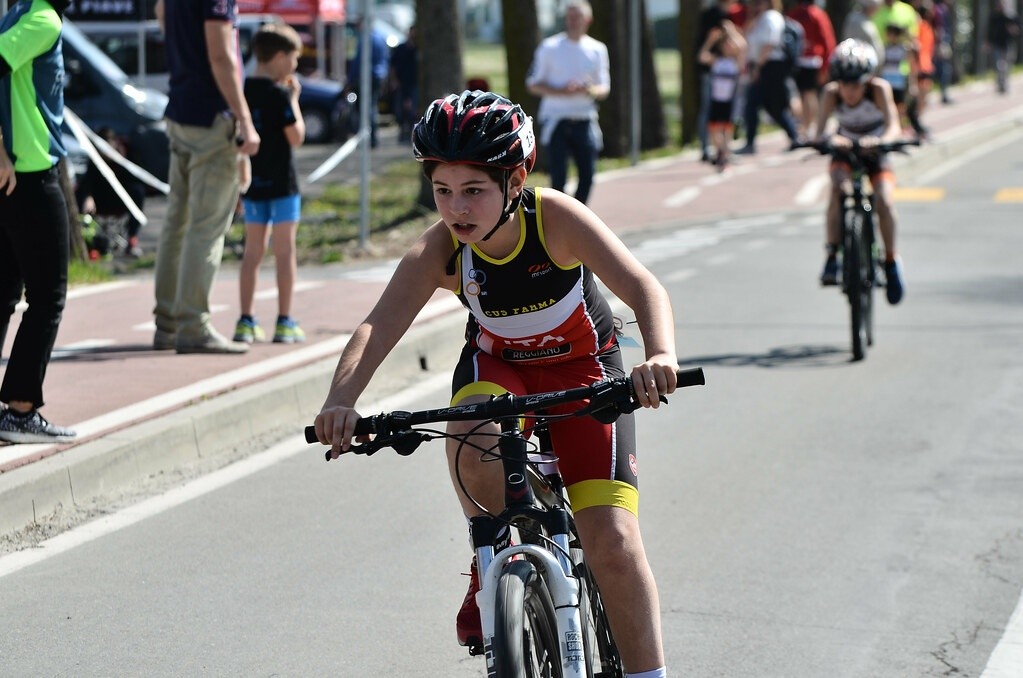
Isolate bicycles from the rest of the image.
[305,365,704,677]
[789,138,919,361]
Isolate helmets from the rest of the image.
[410,91,537,174]
[828,38,879,81]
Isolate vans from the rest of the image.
[61,18,172,186]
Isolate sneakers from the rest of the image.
[154,329,179,351]
[176,330,251,353]
[273,321,306,345]
[0,406,76,444]
[234,323,264,342]
[456,541,513,647]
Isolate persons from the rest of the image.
[74,128,144,258]
[389,26,420,144]
[150,1,306,356]
[812,38,904,306]
[0,0,76,442]
[315,91,680,678]
[339,17,390,149]
[525,0,612,203]
[693,0,1020,176]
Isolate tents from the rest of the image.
[234,0,345,80]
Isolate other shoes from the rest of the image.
[820,254,836,286]
[885,262,904,305]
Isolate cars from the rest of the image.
[298,78,350,146]
